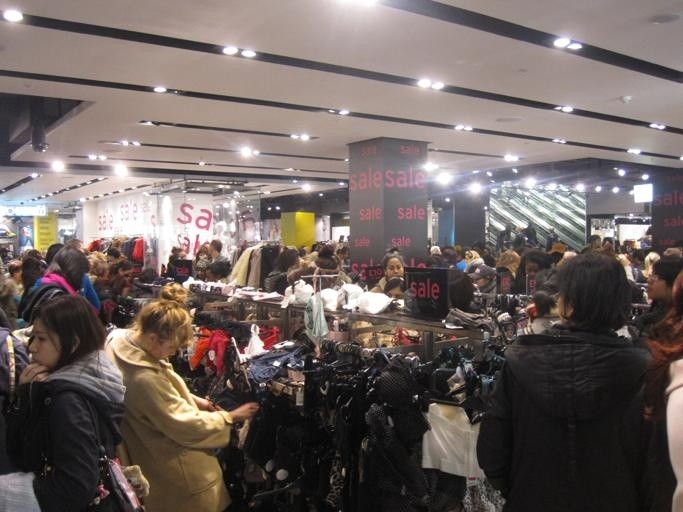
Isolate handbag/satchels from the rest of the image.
[38,389,144,512]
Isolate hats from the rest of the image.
[467,263,495,280]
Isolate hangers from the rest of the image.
[479,293,528,325]
[250,241,286,254]
[194,305,252,346]
[303,337,513,411]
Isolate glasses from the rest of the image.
[648,274,664,282]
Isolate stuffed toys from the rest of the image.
[123,466,150,499]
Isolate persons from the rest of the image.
[19,226,34,246]
[476,251,677,512]
[645,268,683,512]
[0,240,231,399]
[0,294,126,511]
[103,281,260,511]
[263,222,681,330]
